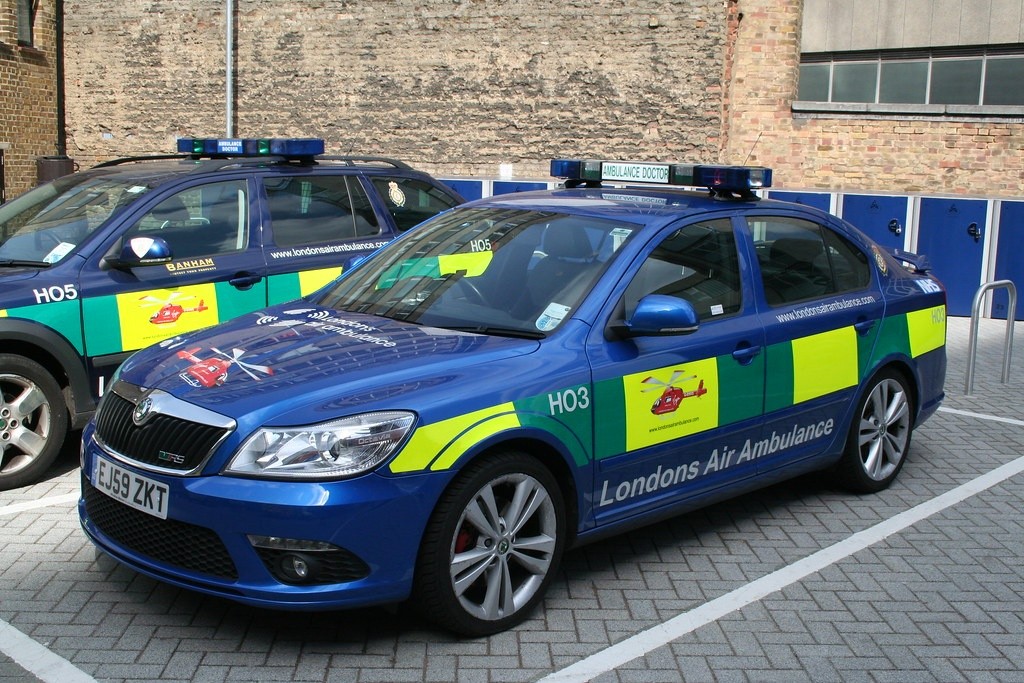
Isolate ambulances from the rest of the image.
[78,158,947,636]
[0,137,471,489]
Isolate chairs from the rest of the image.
[505,218,605,326]
[182,202,254,261]
[612,236,735,330]
[125,194,194,261]
